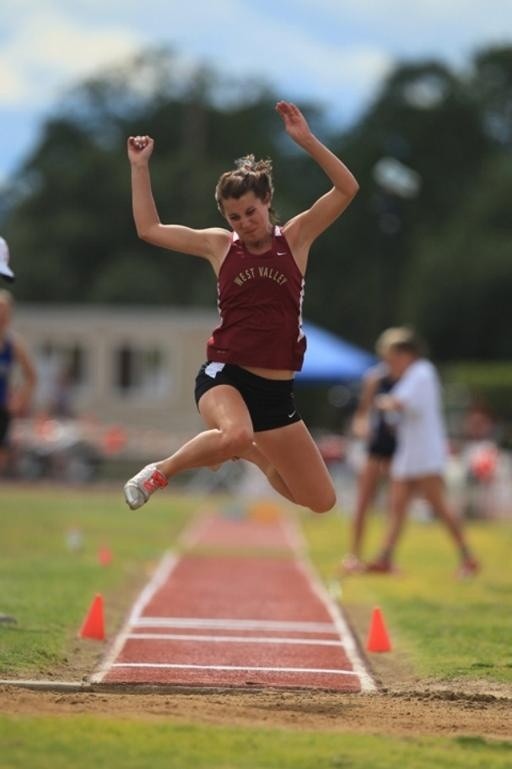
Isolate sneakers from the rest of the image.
[124,462,168,511]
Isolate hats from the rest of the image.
[0,231,14,280]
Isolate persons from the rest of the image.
[124,101,360,513]
[365,325,480,577]
[345,363,422,572]
[0,288,35,480]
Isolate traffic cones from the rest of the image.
[367,607,391,652]
[79,594,104,640]
